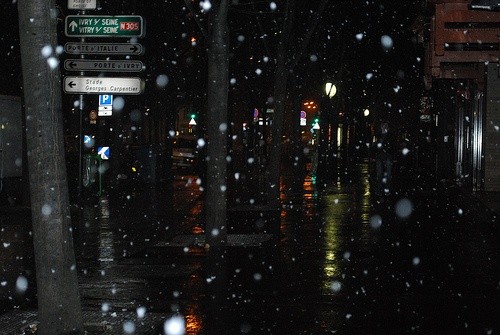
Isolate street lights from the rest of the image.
[324,81,337,166]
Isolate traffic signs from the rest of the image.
[65,15,143,37]
[63,59,143,72]
[63,76,143,95]
[63,43,143,55]
